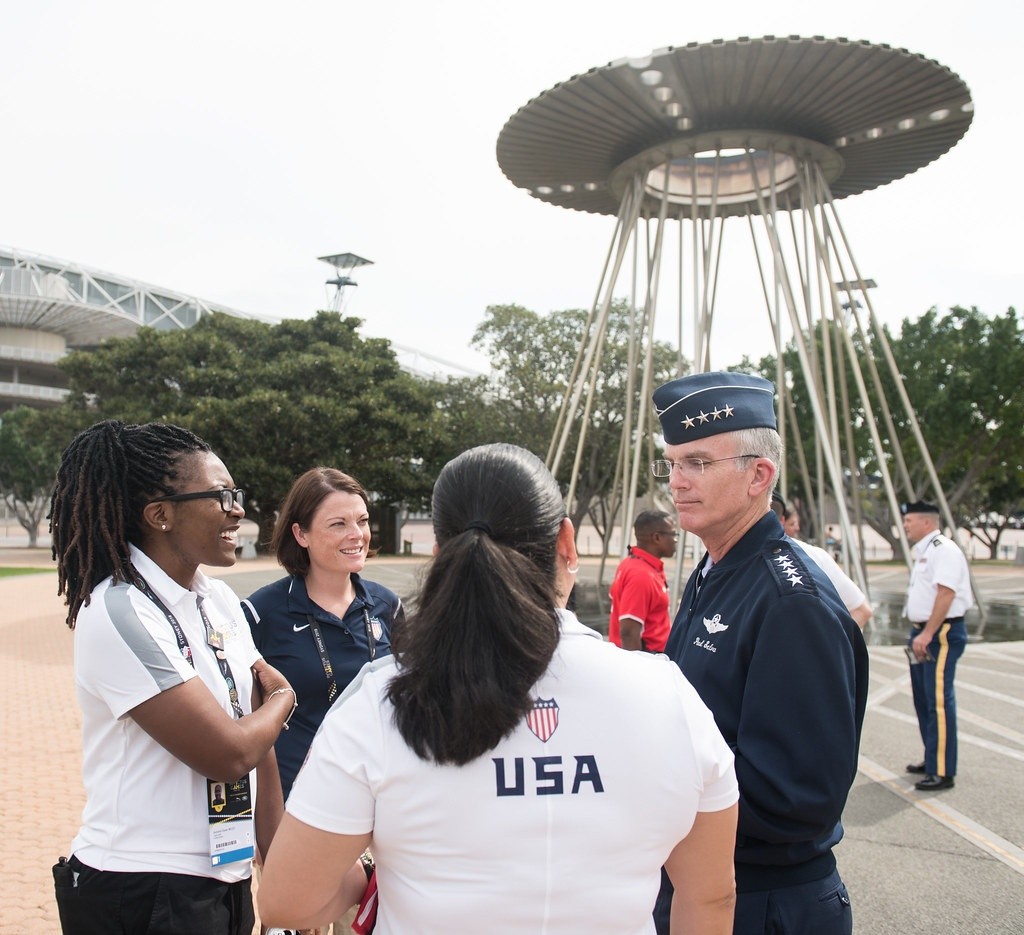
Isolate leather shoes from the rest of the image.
[907,762,927,774]
[914,775,954,790]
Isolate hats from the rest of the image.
[652,372,777,445]
[772,491,786,514]
[900,500,940,515]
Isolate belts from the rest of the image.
[912,617,964,630]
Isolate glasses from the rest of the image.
[650,455,761,478]
[652,531,680,541]
[147,489,245,512]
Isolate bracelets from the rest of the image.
[268,689,298,730]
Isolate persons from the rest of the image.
[257,444,741,935]
[652,372,869,935]
[608,510,680,654]
[240,467,407,935]
[212,785,225,806]
[47,419,298,935]
[768,490,873,627]
[899,501,974,791]
